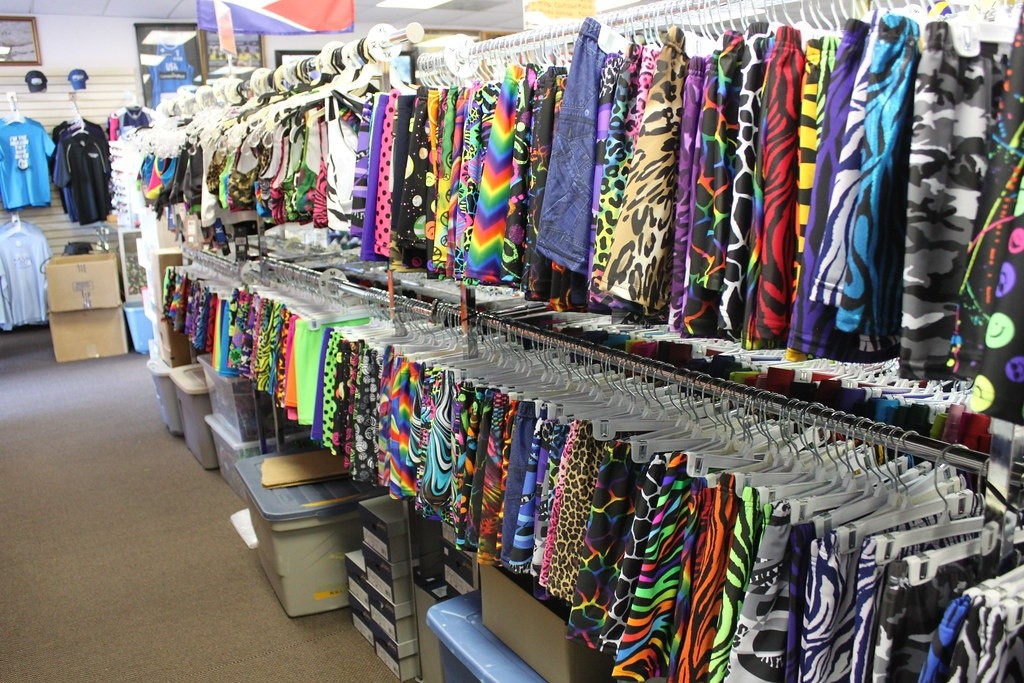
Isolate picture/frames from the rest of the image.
[0,15,42,65]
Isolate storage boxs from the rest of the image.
[124,300,154,355]
[193,352,309,442]
[147,356,183,433]
[343,549,371,609]
[45,252,122,312]
[46,306,129,362]
[372,620,421,682]
[360,542,440,602]
[441,521,478,558]
[479,561,617,683]
[347,591,375,649]
[359,495,441,561]
[369,587,416,644]
[156,307,191,368]
[441,538,478,595]
[429,590,549,683]
[202,414,313,500]
[151,251,183,308]
[233,444,388,621]
[171,362,220,470]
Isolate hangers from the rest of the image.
[126,91,143,111]
[69,101,89,137]
[2,96,25,125]
[180,0,1024,607]
[4,210,31,238]
